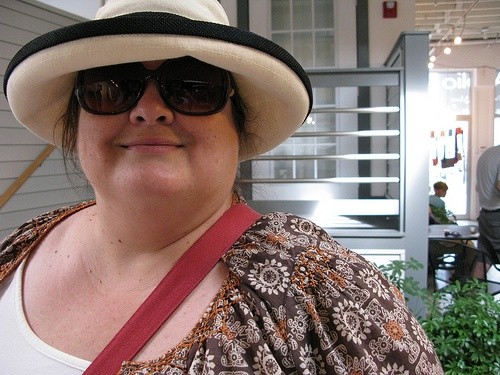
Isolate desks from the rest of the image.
[429,240,500,297]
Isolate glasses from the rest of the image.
[75,55,235,116]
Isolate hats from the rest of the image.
[4,0,313,163]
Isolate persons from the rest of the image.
[429,180,476,285]
[469,145,500,279]
[0,1,445,375]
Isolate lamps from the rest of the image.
[427,23,462,68]
